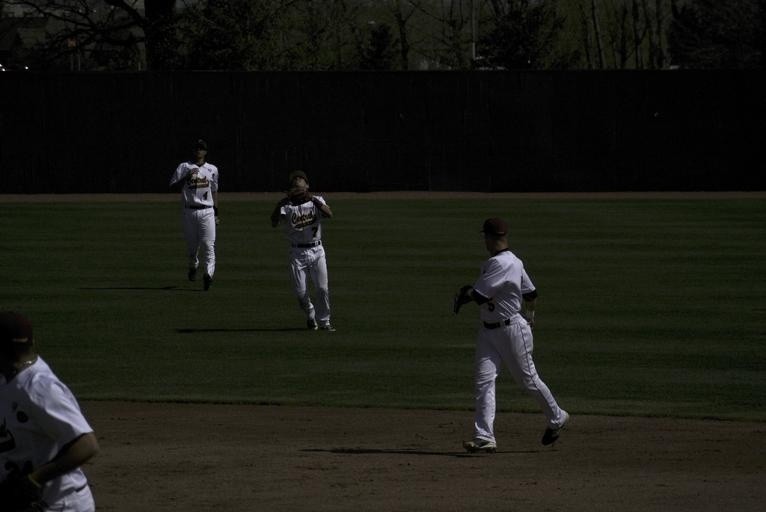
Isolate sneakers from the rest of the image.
[189,266,198,280]
[462,437,498,452]
[306,317,337,333]
[203,274,213,292]
[541,410,569,446]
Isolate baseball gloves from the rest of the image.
[454,286,474,314]
[289,186,313,205]
[1,470,42,512]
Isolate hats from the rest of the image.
[290,170,308,184]
[478,217,508,236]
[193,138,209,152]
[1,311,34,348]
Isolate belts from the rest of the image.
[291,241,320,249]
[482,318,512,331]
[187,204,210,210]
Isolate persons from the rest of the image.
[270,168,339,334]
[167,137,225,291]
[450,215,570,453]
[1,303,101,512]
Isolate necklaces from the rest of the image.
[9,359,37,377]
